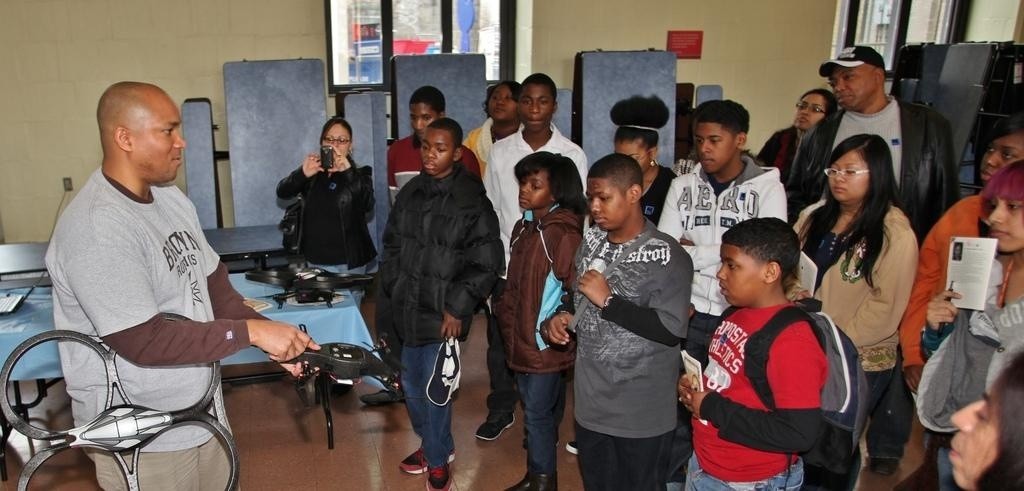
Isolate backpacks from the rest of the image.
[743,298,872,489]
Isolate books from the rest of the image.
[943,235,999,312]
[681,348,708,426]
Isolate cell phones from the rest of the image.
[321,146,333,167]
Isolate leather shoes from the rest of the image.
[870,457,899,474]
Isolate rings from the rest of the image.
[310,157,314,161]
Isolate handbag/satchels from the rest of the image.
[278,200,303,255]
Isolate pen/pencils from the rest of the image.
[937,289,954,337]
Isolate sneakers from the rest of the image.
[474,410,516,441]
[420,444,452,490]
[398,442,456,474]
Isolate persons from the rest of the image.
[947,344,1024,491]
[490,151,589,490]
[898,110,1024,395]
[564,89,680,455]
[376,115,507,490]
[655,97,790,490]
[276,115,375,308]
[675,216,828,490]
[475,71,588,442]
[460,78,525,183]
[893,155,1024,491]
[42,79,321,491]
[784,43,961,478]
[757,87,839,227]
[540,150,695,490]
[791,132,921,490]
[360,84,484,405]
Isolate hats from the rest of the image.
[424,335,462,406]
[818,46,885,78]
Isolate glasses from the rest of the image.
[322,136,349,147]
[823,167,865,181]
[795,102,825,115]
